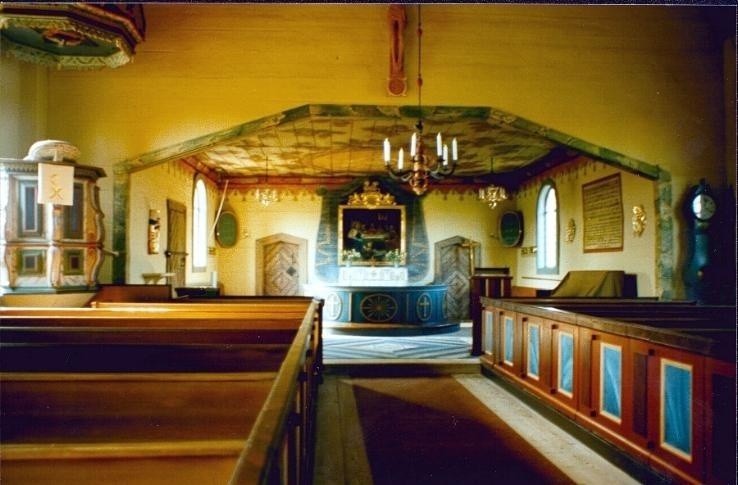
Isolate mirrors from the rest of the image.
[498,209,522,247]
[216,211,239,248]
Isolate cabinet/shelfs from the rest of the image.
[1,157,107,291]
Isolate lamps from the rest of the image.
[383,1,459,198]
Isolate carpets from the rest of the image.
[351,372,579,485]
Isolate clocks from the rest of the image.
[676,177,719,302]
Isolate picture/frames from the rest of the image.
[337,204,407,267]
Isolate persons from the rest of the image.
[347,221,397,259]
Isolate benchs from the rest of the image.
[0,293,324,485]
[571,305,737,331]
